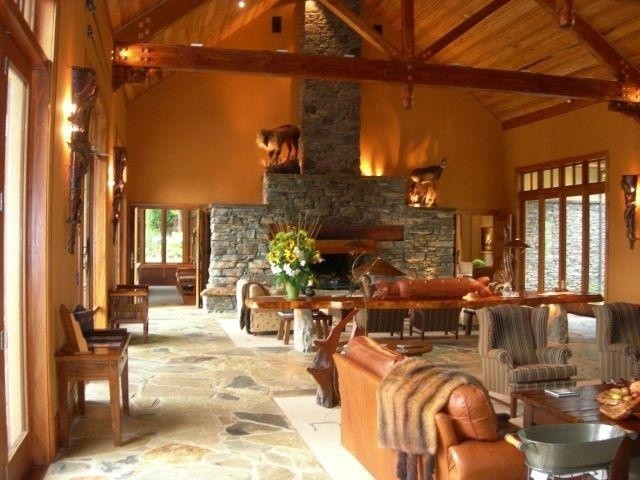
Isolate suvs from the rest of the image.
[503,421,637,480]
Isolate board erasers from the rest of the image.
[621,386,631,403]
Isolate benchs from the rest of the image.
[175,262,196,296]
[109,284,149,338]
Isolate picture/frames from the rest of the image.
[56,328,130,448]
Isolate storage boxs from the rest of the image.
[109,284,149,338]
[175,262,196,296]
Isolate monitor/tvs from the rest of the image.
[544,387,579,397]
[86,328,127,347]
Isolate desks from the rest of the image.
[56,328,130,448]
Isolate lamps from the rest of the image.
[352,254,407,281]
[504,238,533,291]
[65,63,98,254]
[619,172,640,251]
[111,145,128,245]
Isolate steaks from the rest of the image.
[630,381,640,391]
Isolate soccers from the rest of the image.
[631,392,640,400]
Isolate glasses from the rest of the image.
[65,63,98,254]
[352,254,407,281]
[111,145,128,245]
[619,172,640,251]
[504,238,533,291]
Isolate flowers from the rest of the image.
[257,209,326,289]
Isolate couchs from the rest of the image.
[235,270,640,356]
[310,298,639,480]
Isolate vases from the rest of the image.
[257,209,326,289]
